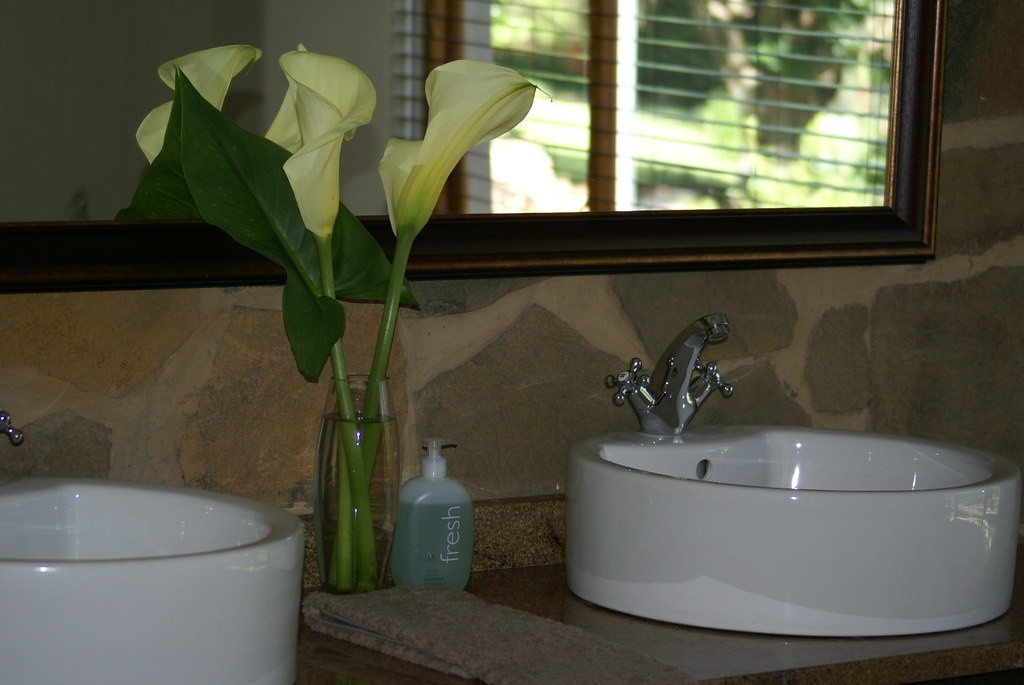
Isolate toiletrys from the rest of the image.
[389,436,476,595]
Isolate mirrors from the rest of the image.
[0,0,950,305]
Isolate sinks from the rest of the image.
[563,421,1023,636]
[1,479,306,685]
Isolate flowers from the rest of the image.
[178,42,550,591]
[106,41,307,223]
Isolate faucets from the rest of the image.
[599,312,734,438]
[0,409,26,450]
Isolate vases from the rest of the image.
[311,376,401,600]
